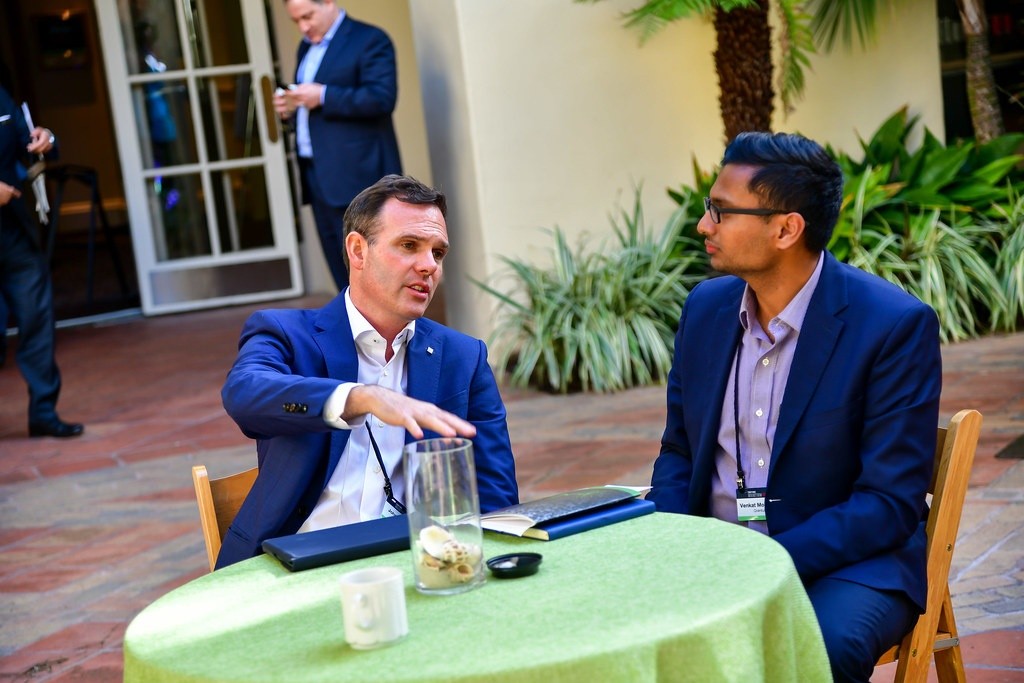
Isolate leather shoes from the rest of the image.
[28,417,84,438]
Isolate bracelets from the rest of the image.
[49,135,55,144]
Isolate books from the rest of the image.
[441,484,656,542]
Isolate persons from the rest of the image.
[135,22,185,192]
[645,132,943,683]
[0,86,84,438]
[213,174,519,573]
[272,0,404,293]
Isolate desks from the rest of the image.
[123,495,839,683]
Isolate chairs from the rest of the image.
[191,455,265,574]
[873,408,984,683]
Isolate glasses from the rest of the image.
[704,197,791,224]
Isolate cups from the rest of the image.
[338,568,410,651]
[402,438,487,595]
